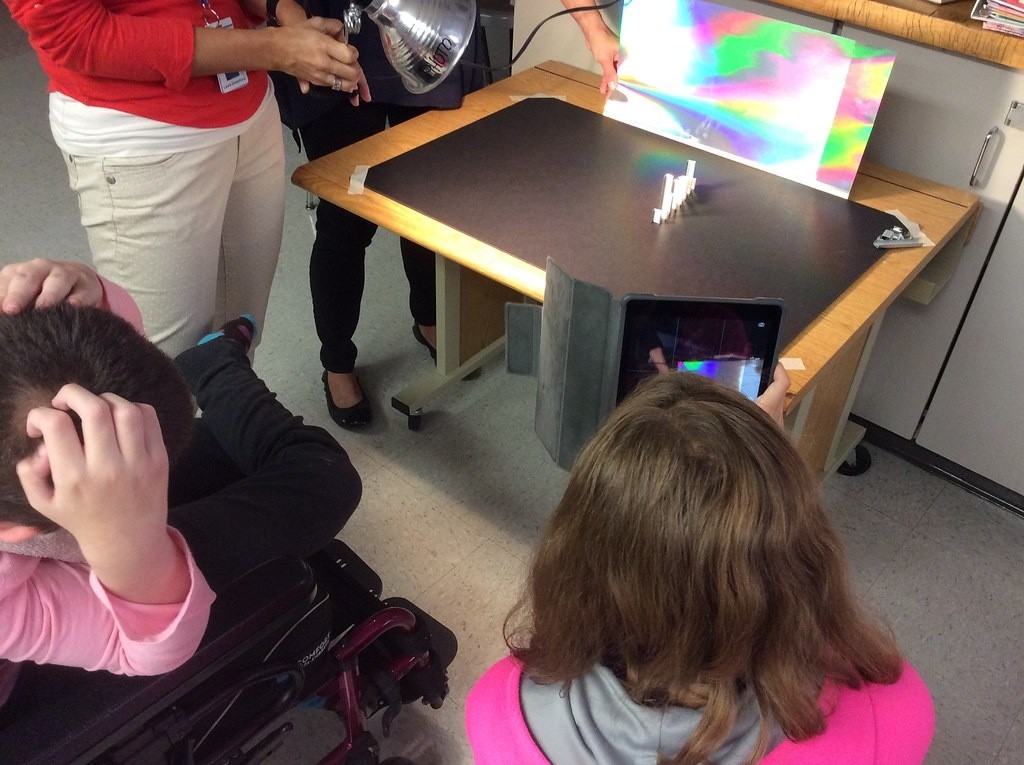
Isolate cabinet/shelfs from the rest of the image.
[510,0,1024,518]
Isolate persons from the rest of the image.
[4,0,371,357]
[0,256,363,702]
[466,362,934,765]
[622,299,669,373]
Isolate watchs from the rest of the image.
[266,0,627,433]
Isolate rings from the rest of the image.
[331,76,342,91]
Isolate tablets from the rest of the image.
[610,294,785,404]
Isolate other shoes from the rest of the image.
[413,320,478,381]
[199,314,254,357]
[322,370,373,431]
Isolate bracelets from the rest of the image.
[267,0,281,27]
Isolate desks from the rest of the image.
[292,57,983,501]
